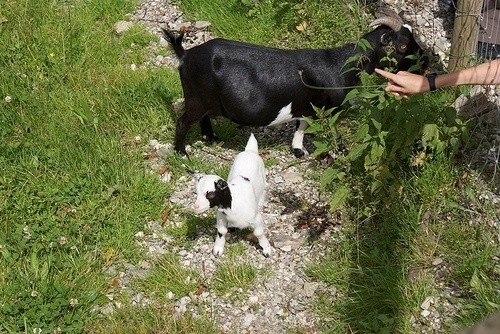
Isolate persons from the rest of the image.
[373,58,499,99]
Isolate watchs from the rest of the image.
[425,73,439,92]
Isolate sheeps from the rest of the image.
[185,132,276,259]
[160,7,430,160]
